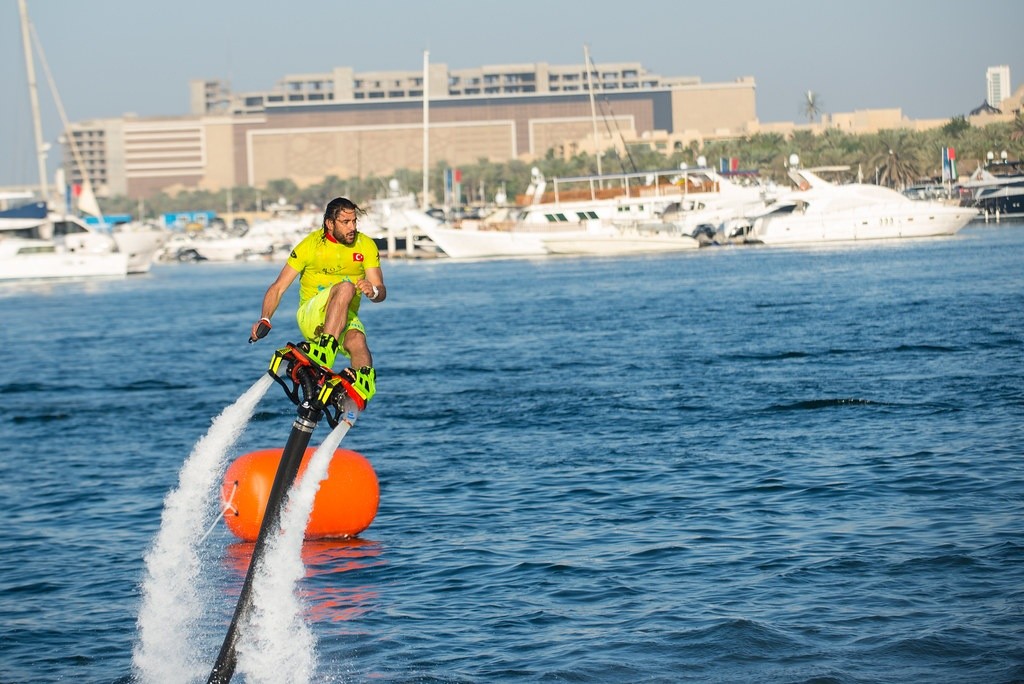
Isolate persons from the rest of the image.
[251,197,386,398]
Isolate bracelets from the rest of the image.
[370,286,379,299]
[257,316,271,326]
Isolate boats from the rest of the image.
[0,0,982,280]
